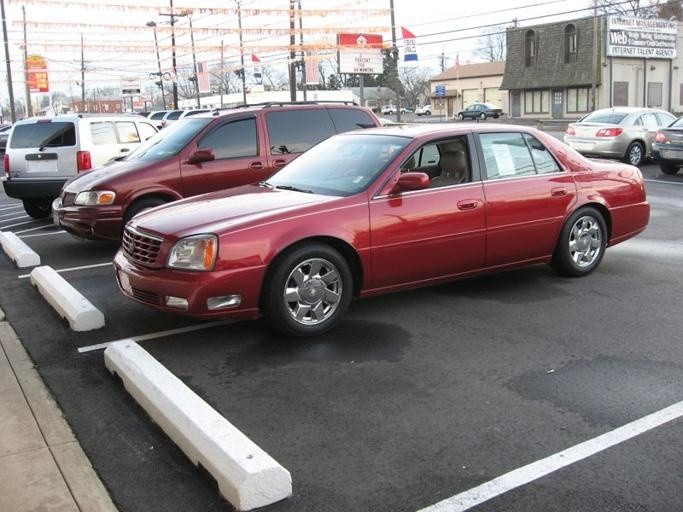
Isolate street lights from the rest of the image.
[145,20,168,109]
[293,59,306,101]
[180,8,201,109]
[232,67,246,105]
[379,46,400,122]
[74,81,85,111]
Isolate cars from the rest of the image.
[378,104,431,116]
[133,109,214,129]
[457,102,504,120]
[110,121,651,342]
[563,106,678,166]
[0,123,11,148]
[652,113,682,175]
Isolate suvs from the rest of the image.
[51,98,382,249]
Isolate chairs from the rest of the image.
[426,149,466,189]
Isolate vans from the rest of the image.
[2,113,159,220]
